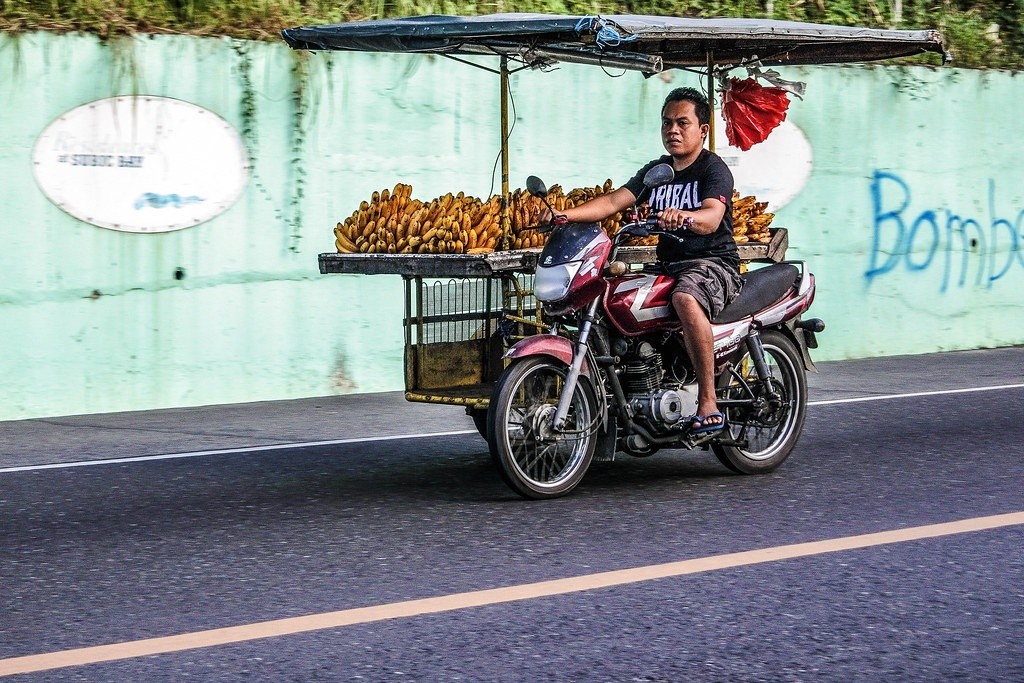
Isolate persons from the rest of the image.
[536,88,745,432]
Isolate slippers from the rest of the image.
[691,412,727,434]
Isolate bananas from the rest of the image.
[330,181,774,254]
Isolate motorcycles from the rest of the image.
[486,163,827,500]
